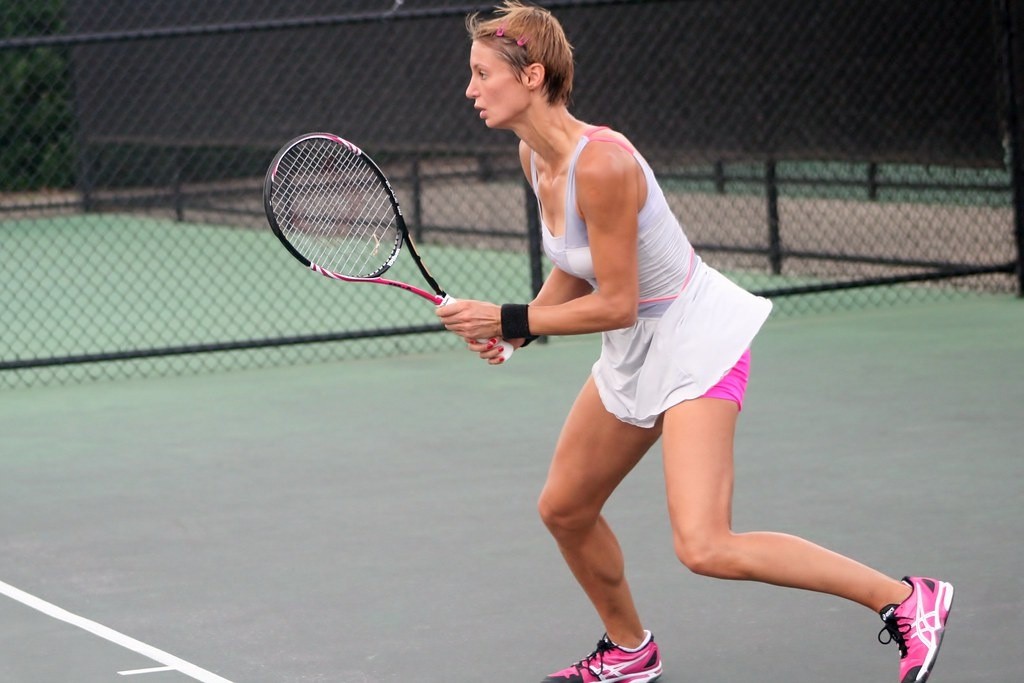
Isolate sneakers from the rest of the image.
[541,630,663,683]
[878,576,954,683]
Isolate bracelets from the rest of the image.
[501,303,531,339]
[521,336,539,347]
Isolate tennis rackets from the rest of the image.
[260,130,514,365]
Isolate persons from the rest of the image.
[435,0,954,683]
